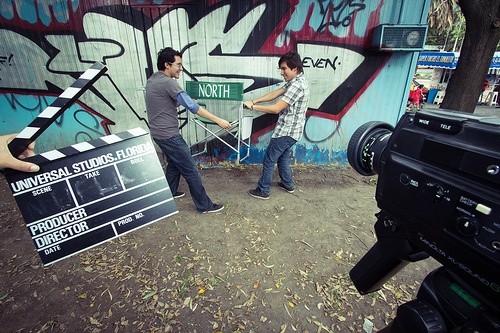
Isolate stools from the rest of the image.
[484,92,498,106]
[433,91,445,104]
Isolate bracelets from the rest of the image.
[252,105,254,110]
[252,101,255,105]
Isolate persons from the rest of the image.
[145,48,231,213]
[406,85,424,111]
[476,79,489,102]
[411,77,416,91]
[243,51,310,199]
[493,78,500,108]
[0,134,40,172]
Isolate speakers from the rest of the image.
[372,23,429,53]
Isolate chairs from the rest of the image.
[186,81,254,165]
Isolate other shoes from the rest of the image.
[198,201,224,213]
[276,181,294,194]
[247,187,270,200]
[172,191,186,199]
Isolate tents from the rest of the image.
[416,51,500,90]
